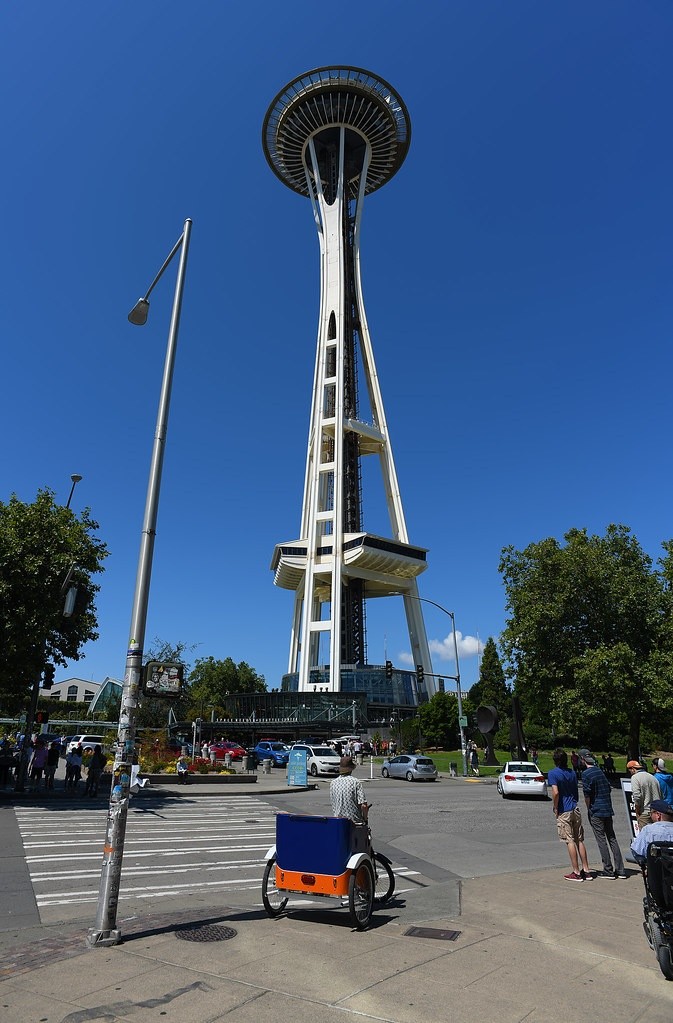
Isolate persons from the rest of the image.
[321,736,489,895]
[0,725,231,800]
[531,748,673,882]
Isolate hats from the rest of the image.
[652,758,668,771]
[340,757,356,771]
[626,760,643,768]
[578,749,595,762]
[648,799,670,814]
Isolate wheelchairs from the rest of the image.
[637,840,673,981]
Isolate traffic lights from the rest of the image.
[385,660,393,679]
[35,712,50,724]
[417,664,424,685]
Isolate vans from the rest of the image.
[67,734,106,755]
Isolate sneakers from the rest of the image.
[614,872,627,878]
[580,869,594,881]
[563,872,583,882]
[597,872,616,879]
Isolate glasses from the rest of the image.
[648,811,657,816]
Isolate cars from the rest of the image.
[284,735,361,755]
[289,743,342,778]
[210,740,249,762]
[495,761,548,801]
[254,741,290,768]
[381,754,437,782]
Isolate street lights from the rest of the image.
[87,211,197,948]
[65,473,83,508]
[386,591,469,778]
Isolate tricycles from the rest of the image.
[261,802,396,932]
